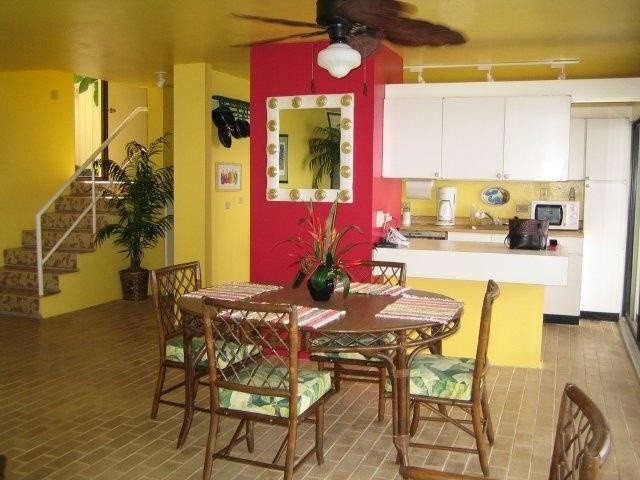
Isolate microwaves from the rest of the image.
[531,200,580,231]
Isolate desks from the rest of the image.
[171,277,463,479]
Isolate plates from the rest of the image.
[481,186,510,206]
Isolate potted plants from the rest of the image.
[99,138,177,302]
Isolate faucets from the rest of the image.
[480,210,499,228]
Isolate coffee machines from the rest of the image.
[436,186,457,226]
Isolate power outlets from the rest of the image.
[385,212,391,224]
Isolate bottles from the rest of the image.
[401,203,411,226]
[469,204,477,225]
[569,187,575,201]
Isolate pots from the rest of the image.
[211,101,250,147]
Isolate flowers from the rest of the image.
[281,195,370,300]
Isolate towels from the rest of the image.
[214,298,347,334]
[375,295,464,326]
[331,278,412,297]
[186,277,285,303]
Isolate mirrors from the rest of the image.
[266,91,355,206]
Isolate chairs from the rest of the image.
[305,257,407,422]
[381,276,500,479]
[199,295,333,479]
[146,260,263,454]
[399,385,613,479]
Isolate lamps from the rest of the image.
[316,24,362,81]
[402,64,579,82]
[153,70,169,89]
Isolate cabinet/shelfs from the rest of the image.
[382,83,443,180]
[584,181,631,322]
[584,115,631,183]
[492,234,582,317]
[437,78,573,181]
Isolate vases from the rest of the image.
[305,273,335,301]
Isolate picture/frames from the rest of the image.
[212,162,243,194]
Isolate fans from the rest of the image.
[218,0,465,80]
